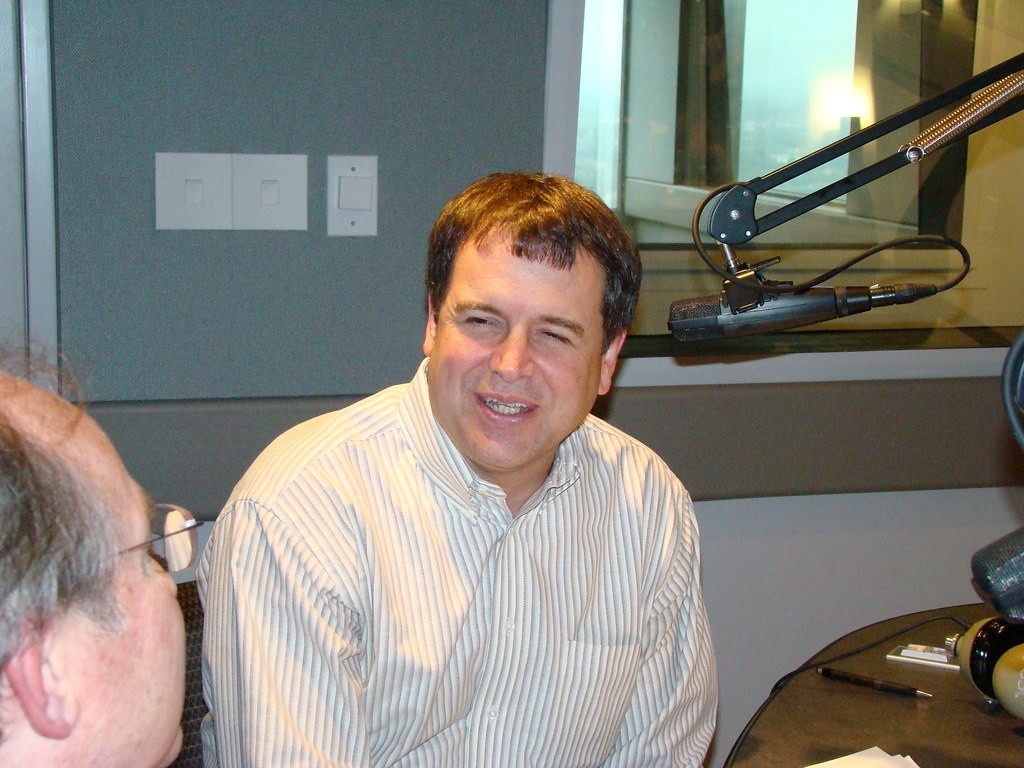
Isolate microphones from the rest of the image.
[972,527,1024,624]
[667,282,942,341]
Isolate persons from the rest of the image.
[0,170,718,768]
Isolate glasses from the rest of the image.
[97,504,204,572]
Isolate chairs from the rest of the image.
[168,581,210,768]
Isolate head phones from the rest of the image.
[945,616,1024,721]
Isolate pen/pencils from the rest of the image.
[816,666,933,699]
